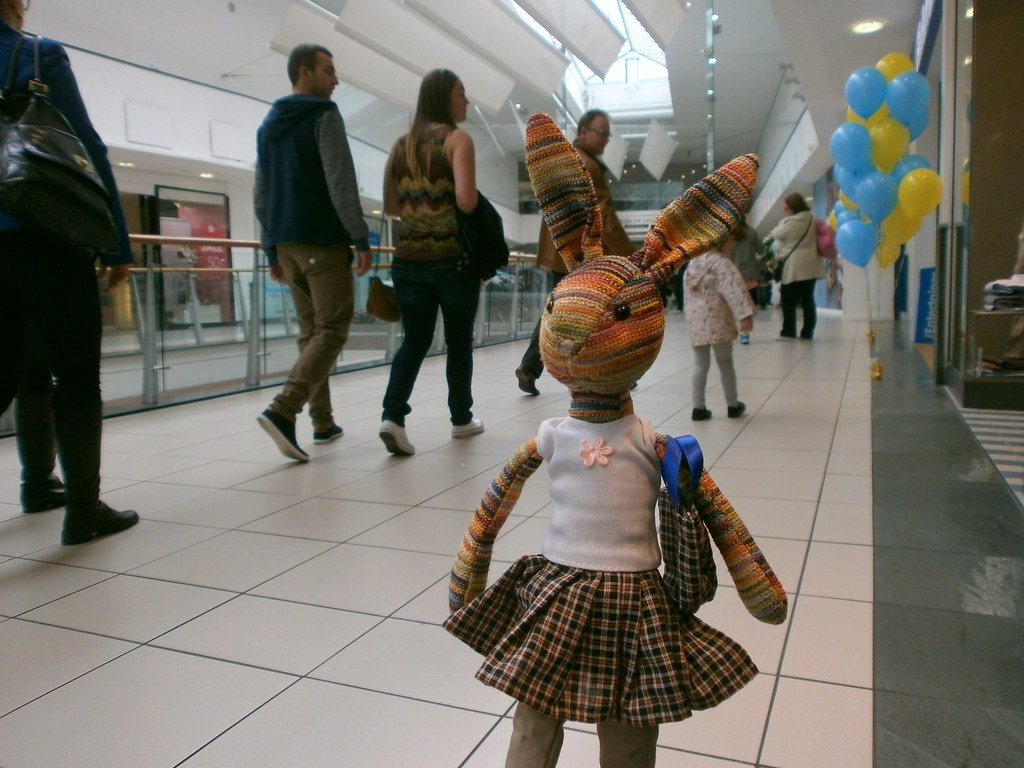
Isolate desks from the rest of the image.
[969,308,1024,376]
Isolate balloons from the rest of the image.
[831,51,945,271]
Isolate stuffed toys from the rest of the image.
[443,108,789,768]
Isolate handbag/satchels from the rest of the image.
[0,33,122,256]
[366,276,401,322]
[817,221,833,260]
[774,262,782,281]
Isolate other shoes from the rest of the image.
[379,419,415,454]
[515,364,540,396]
[451,418,484,439]
[313,422,343,444]
[692,407,712,420]
[728,402,745,417]
[257,409,310,462]
[740,330,750,344]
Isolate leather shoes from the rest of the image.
[61,499,139,545]
[19,473,67,514]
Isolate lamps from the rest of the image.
[779,62,805,102]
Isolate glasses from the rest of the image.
[10,0,30,10]
[585,127,613,138]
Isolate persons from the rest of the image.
[0,0,139,548]
[725,213,772,344]
[770,192,825,340]
[649,262,689,312]
[379,68,483,455]
[514,108,636,396]
[255,45,372,461]
[683,224,757,420]
